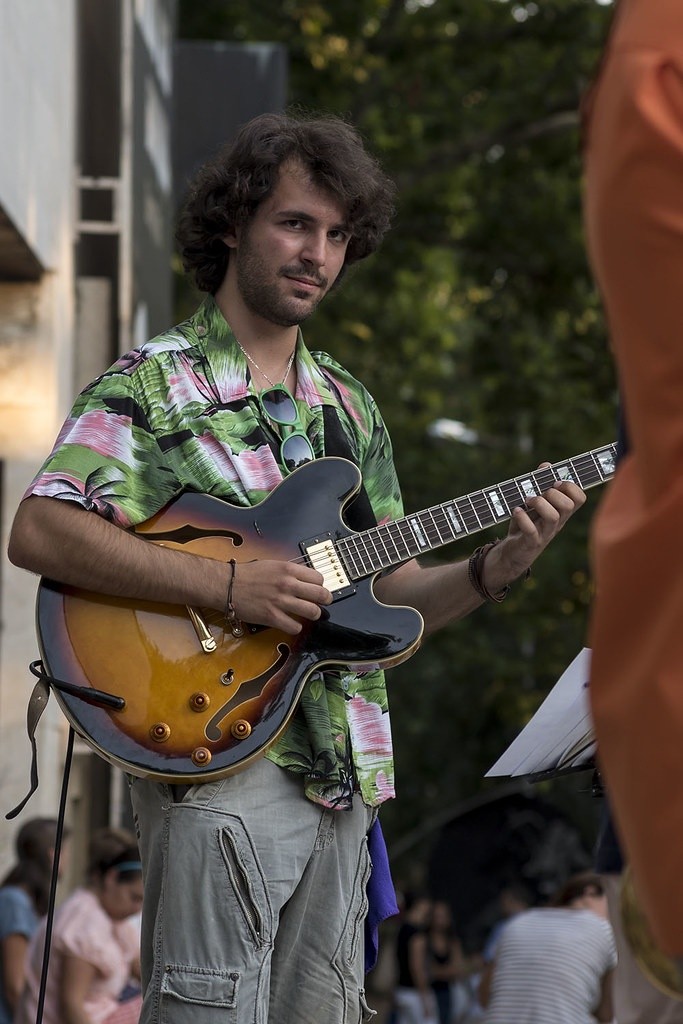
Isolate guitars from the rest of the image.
[34,437,622,782]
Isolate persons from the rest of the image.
[0,819,144,1024]
[403,821,630,1024]
[6,113,587,1024]
[584,0,683,1024]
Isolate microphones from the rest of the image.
[29,659,126,711]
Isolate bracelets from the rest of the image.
[225,558,238,620]
[468,540,513,603]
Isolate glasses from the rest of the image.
[260,381,315,475]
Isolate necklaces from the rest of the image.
[236,341,295,387]
